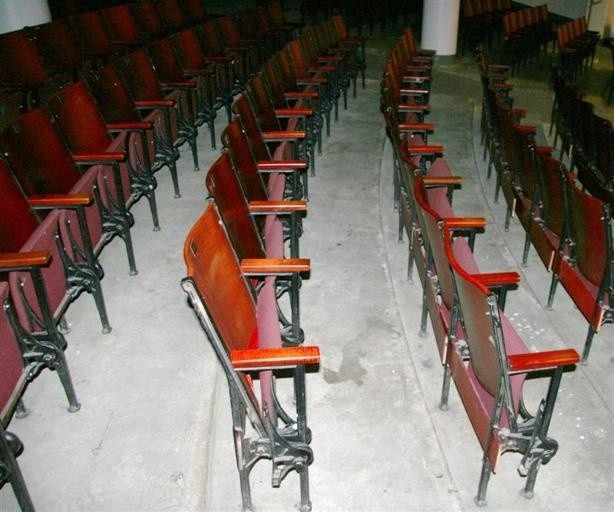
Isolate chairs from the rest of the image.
[0,0,614,512]
